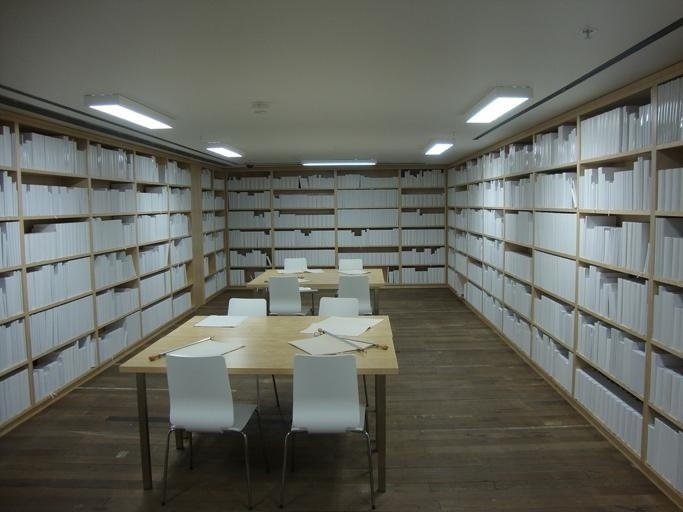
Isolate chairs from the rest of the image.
[243,258,387,314]
[225,298,281,418]
[279,354,376,509]
[161,354,266,512]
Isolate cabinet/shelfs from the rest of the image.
[0,110,227,437]
[228,166,445,290]
[447,57,682,510]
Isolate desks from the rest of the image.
[120,315,397,493]
